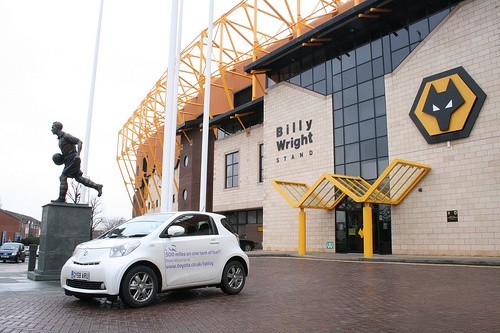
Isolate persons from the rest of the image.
[51,121,104,202]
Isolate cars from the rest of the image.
[59,211,250,308]
[0,243,27,263]
